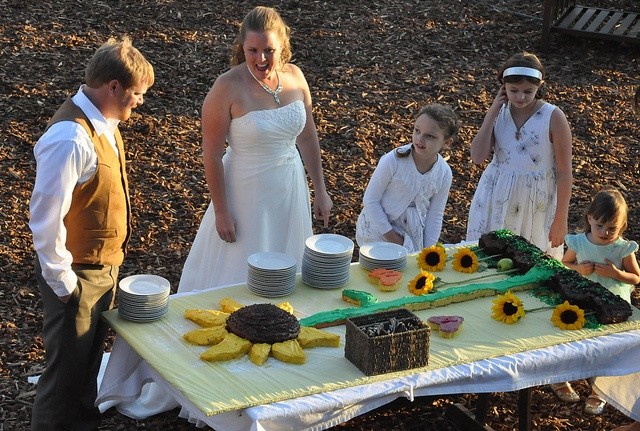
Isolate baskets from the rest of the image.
[344,308,432,377]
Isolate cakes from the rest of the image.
[427,315,465,339]
[182,229,632,369]
[368,268,402,293]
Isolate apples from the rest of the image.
[497,258,514,272]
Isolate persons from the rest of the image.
[559,190,640,303]
[175,5,333,294]
[467,52,571,261]
[356,103,460,254]
[28,34,155,431]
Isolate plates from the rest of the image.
[302,233,354,290]
[360,242,408,272]
[246,251,297,299]
[119,275,172,323]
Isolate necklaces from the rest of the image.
[511,98,538,141]
[245,62,286,104]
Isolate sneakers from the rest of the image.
[549,383,582,402]
[585,391,606,415]
